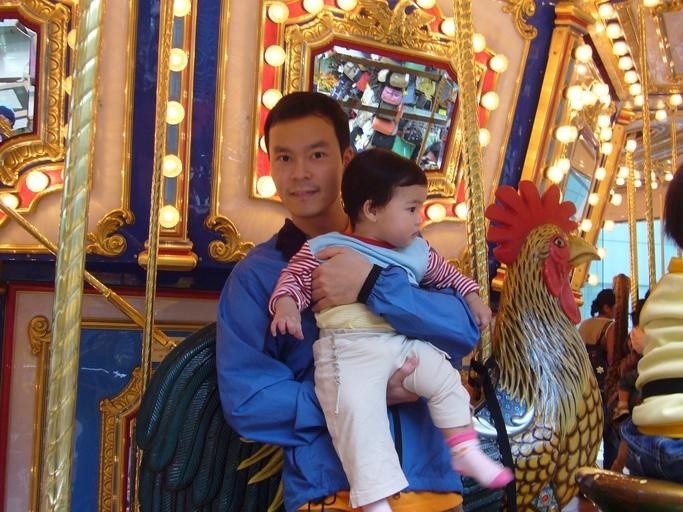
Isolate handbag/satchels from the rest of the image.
[584,343,608,392]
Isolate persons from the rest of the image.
[264,145,515,510]
[577,286,654,473]
[618,163,681,483]
[214,89,482,512]
